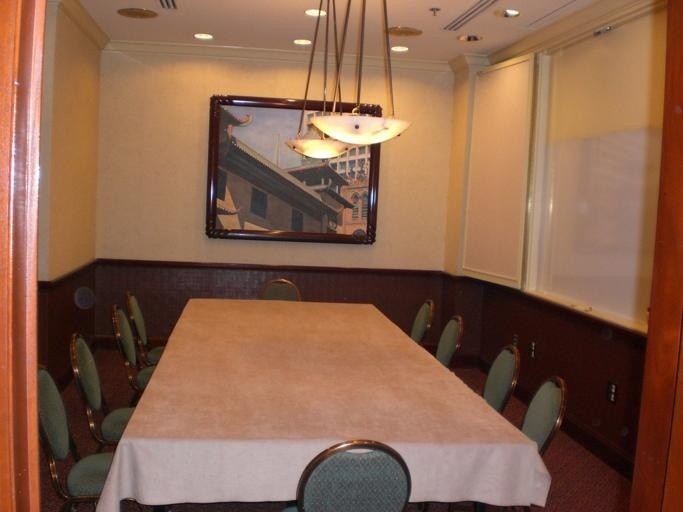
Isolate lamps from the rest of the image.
[284,0,356,160]
[307,0,412,145]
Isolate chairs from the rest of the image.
[410,298,570,456]
[259,278,302,302]
[295,439,412,512]
[38,289,167,512]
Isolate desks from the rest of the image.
[94,297,552,512]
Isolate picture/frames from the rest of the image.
[205,94,383,245]
